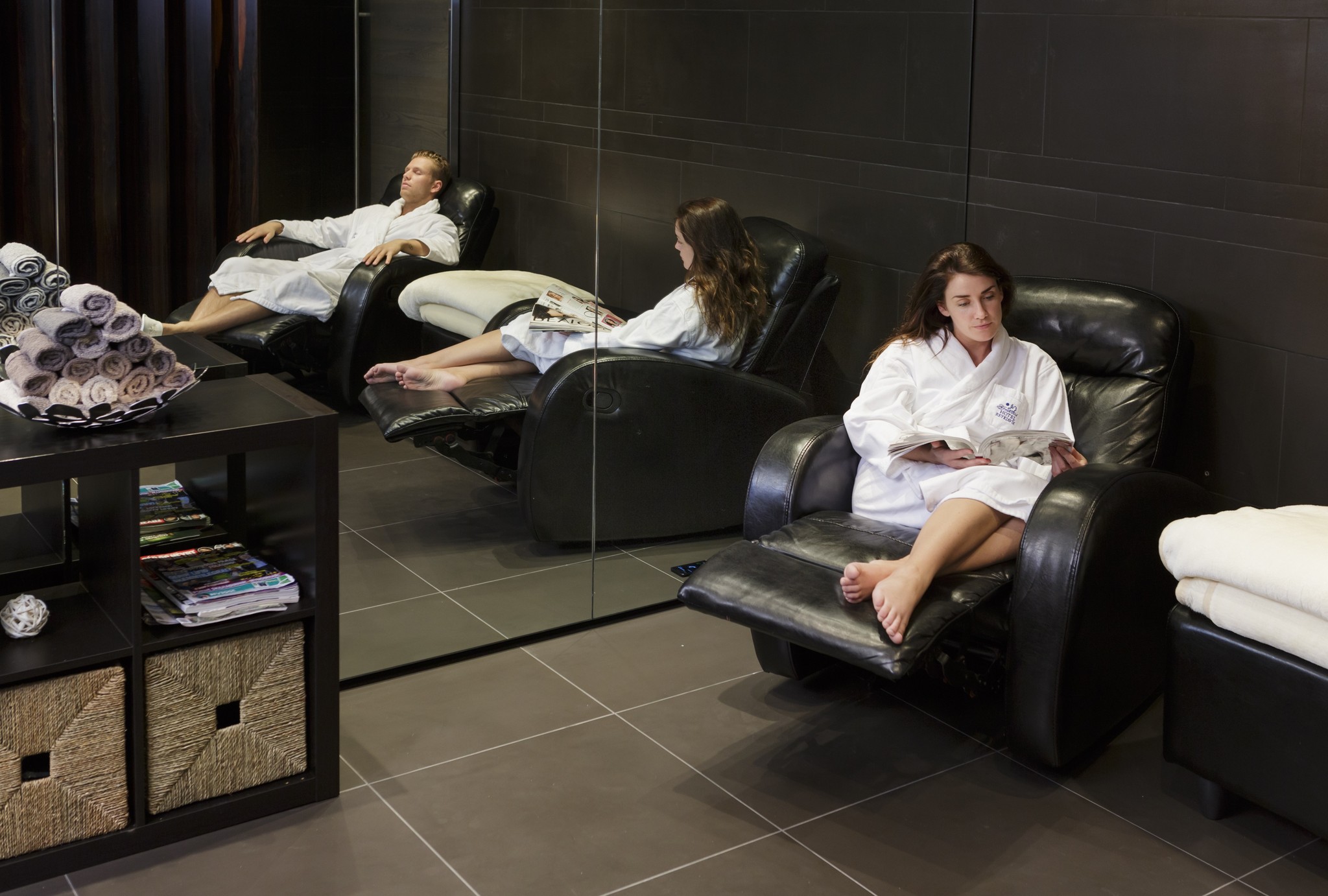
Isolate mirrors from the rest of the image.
[0,1,977,689]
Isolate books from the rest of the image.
[139,541,300,628]
[887,430,1073,464]
[69,480,211,547]
[529,284,626,333]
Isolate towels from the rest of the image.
[16,328,75,371]
[0,380,181,428]
[1158,505,1328,670]
[97,349,132,380]
[0,262,30,295]
[117,366,155,406]
[60,283,117,325]
[119,331,154,362]
[5,350,57,397]
[0,242,48,282]
[82,374,118,407]
[33,261,71,291]
[161,362,195,387]
[0,285,75,363]
[101,300,142,342]
[72,328,111,359]
[60,358,97,385]
[49,377,82,406]
[145,337,176,386]
[33,307,92,346]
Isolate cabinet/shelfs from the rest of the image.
[1,331,249,595]
[0,372,335,893]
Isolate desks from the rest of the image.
[421,323,469,353]
[1164,596,1327,843]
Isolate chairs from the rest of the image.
[676,279,1191,775]
[363,214,845,544]
[170,169,502,415]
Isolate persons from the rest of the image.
[137,149,460,336]
[199,548,216,554]
[238,553,268,569]
[156,559,182,571]
[572,297,584,303]
[587,307,603,316]
[183,510,202,519]
[166,491,196,509]
[532,304,575,319]
[363,196,768,391]
[605,318,619,327]
[224,544,237,553]
[231,568,246,573]
[548,291,563,301]
[840,242,1089,643]
[165,514,178,521]
